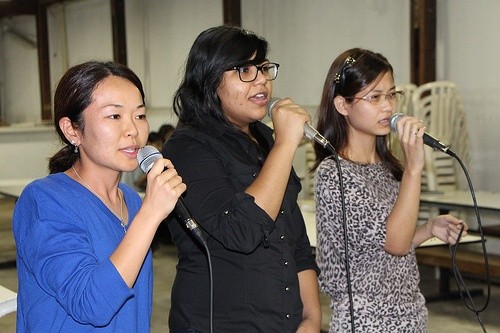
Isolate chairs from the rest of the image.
[385,81,472,279]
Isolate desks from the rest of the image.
[420,191,500,215]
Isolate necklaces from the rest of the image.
[72,165,128,235]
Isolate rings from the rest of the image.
[410,131,416,135]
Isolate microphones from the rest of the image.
[136,145,206,244]
[389,113,456,157]
[268,97,335,153]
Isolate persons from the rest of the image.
[315,48,468,333]
[158,26,323,333]
[13,60,185,333]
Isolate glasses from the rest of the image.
[345,91,405,106]
[224,63,280,82]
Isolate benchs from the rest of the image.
[414,232,500,303]
